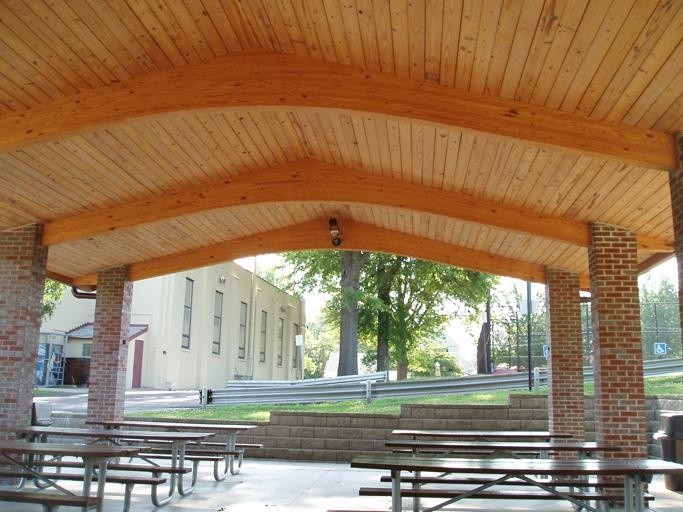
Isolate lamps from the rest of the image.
[328,218,341,246]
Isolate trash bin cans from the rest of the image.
[28,401,52,480]
[660,415,683,492]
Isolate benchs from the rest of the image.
[0,438,263,512]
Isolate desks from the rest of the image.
[0,420,257,506]
[384,429,621,510]
